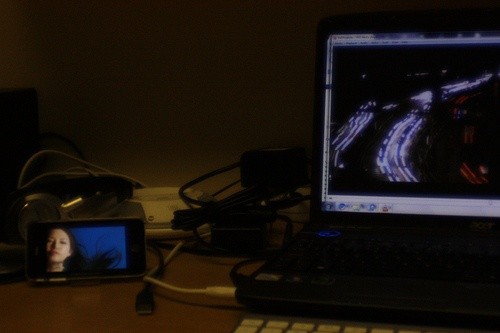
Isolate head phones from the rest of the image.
[4,174,134,246]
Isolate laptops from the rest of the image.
[236,4,500,333]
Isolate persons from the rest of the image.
[46,228,121,274]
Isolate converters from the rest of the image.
[240,145,309,195]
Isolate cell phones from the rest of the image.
[27,216,146,282]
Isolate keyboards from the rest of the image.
[234,314,434,333]
